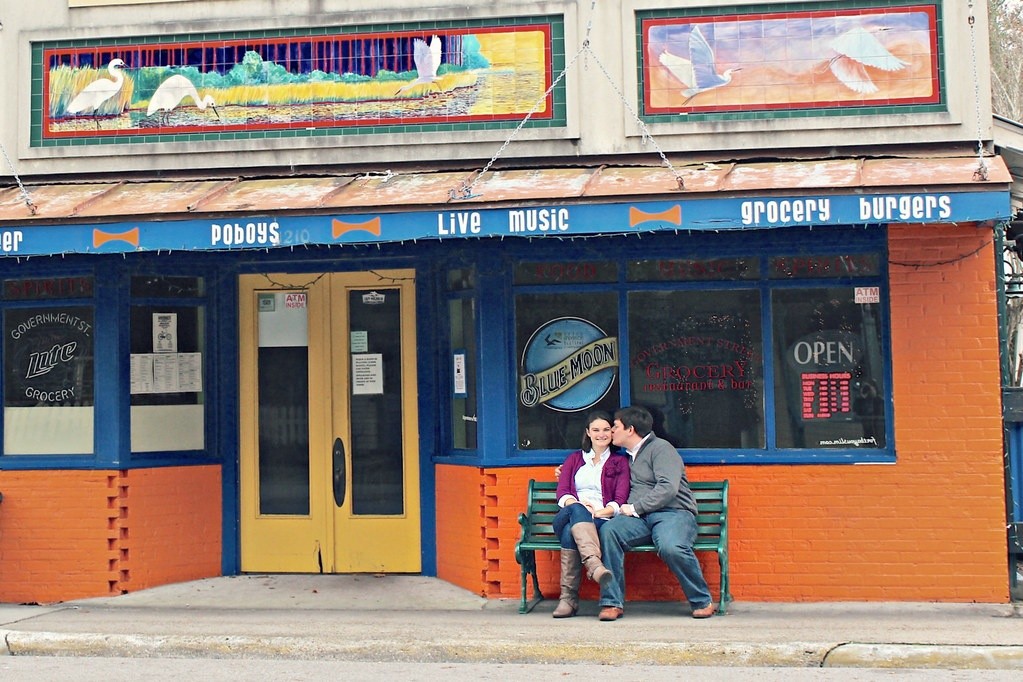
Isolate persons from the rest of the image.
[552,410,631,618]
[555,406,714,620]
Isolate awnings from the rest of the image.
[0,147,1014,259]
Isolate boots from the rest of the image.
[571,522,612,584]
[552,548,582,618]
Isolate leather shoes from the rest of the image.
[692,601,714,618]
[599,607,623,621]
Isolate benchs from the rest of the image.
[513,479,735,616]
